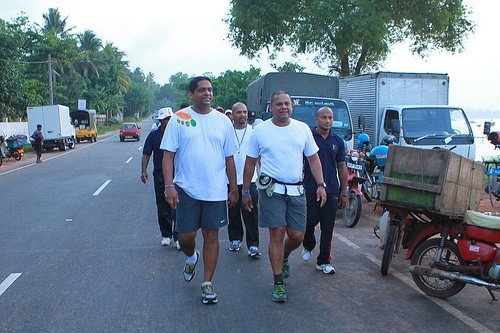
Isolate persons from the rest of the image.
[180,102,189,109]
[140,108,181,250]
[160,77,240,304]
[31,125,44,163]
[370,135,394,186]
[302,107,349,274]
[152,114,161,130]
[215,102,264,255]
[242,90,327,303]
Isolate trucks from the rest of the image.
[25,104,79,153]
[338,70,476,162]
[246,70,367,152]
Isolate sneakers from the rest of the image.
[248,245,262,257]
[174,239,181,249]
[201,280,219,304]
[282,258,291,279]
[182,249,200,282]
[301,247,311,261]
[161,237,171,245]
[228,239,242,251]
[316,262,336,273]
[272,281,288,302]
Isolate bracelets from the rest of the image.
[242,188,249,195]
[164,185,175,197]
[341,193,348,197]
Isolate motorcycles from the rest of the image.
[378,204,500,303]
[70,109,99,143]
[342,148,413,277]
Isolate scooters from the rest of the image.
[0,135,25,161]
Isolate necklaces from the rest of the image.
[234,122,247,154]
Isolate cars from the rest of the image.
[118,122,142,142]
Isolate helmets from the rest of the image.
[380,135,398,145]
[357,133,370,147]
[369,144,389,167]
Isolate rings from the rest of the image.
[346,203,348,204]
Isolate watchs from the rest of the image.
[318,183,326,188]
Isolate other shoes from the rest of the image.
[36,159,42,163]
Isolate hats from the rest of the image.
[153,106,174,120]
[225,108,232,114]
